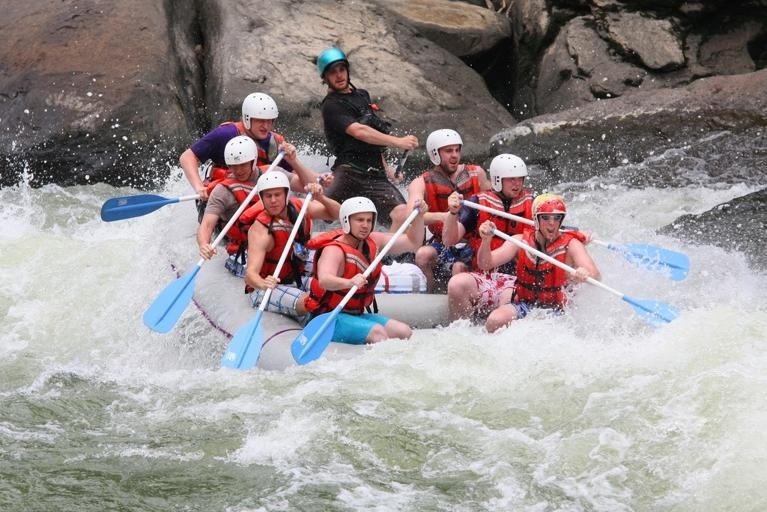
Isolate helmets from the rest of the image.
[317,48,349,77]
[224,136,259,174]
[425,128,464,165]
[241,92,279,130]
[489,153,528,193]
[531,193,567,230]
[256,171,291,208]
[339,196,378,235]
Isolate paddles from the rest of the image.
[492,228,680,327]
[221,193,311,369]
[143,152,286,333]
[290,208,419,365]
[461,200,689,281]
[101,194,200,221]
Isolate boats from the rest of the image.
[163,176,593,386]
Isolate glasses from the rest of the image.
[540,214,563,221]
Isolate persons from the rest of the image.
[316,48,419,226]
[446,193,603,335]
[179,93,335,234]
[243,170,341,318]
[406,128,492,294]
[196,135,316,277]
[303,196,429,345]
[442,153,592,293]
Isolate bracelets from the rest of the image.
[451,212,459,214]
